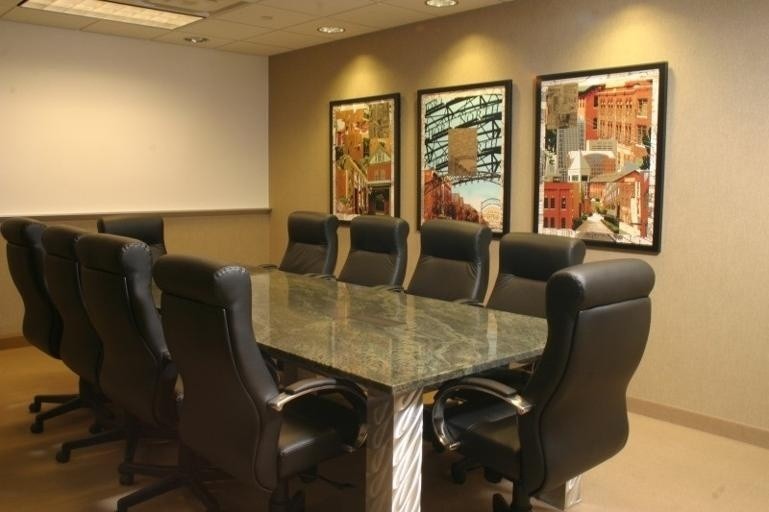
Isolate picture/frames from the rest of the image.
[532,60,668,253]
[330,92,400,219]
[417,79,512,240]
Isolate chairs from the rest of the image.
[277,208,337,280]
[40,223,182,486]
[94,212,170,256]
[338,215,410,289]
[377,218,492,304]
[150,253,367,508]
[431,258,654,512]
[75,231,236,511]
[457,233,588,318]
[0,215,131,436]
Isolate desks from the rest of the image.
[243,264,549,511]
